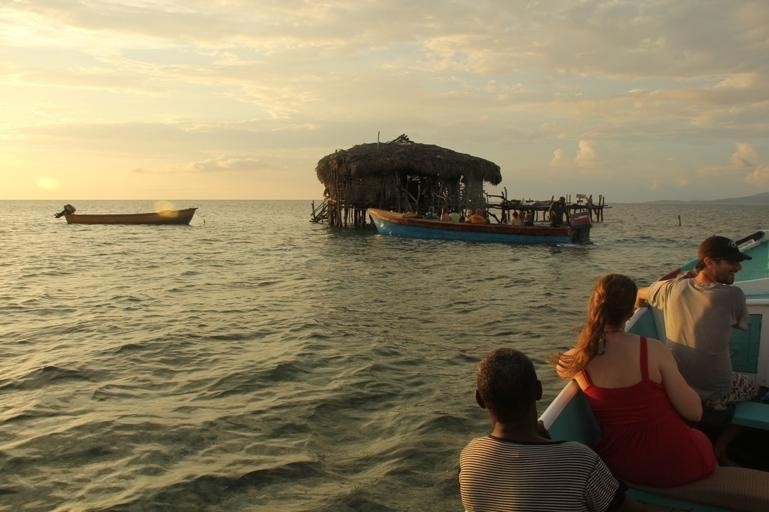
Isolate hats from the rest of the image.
[698,235,752,261]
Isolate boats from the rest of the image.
[364,208,594,245]
[63,202,200,225]
[536,230,768,501]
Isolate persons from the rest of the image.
[456,347,647,512]
[633,235,767,468]
[402,195,571,228]
[549,273,719,488]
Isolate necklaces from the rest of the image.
[605,326,626,335]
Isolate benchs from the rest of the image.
[625,400,769,512]
